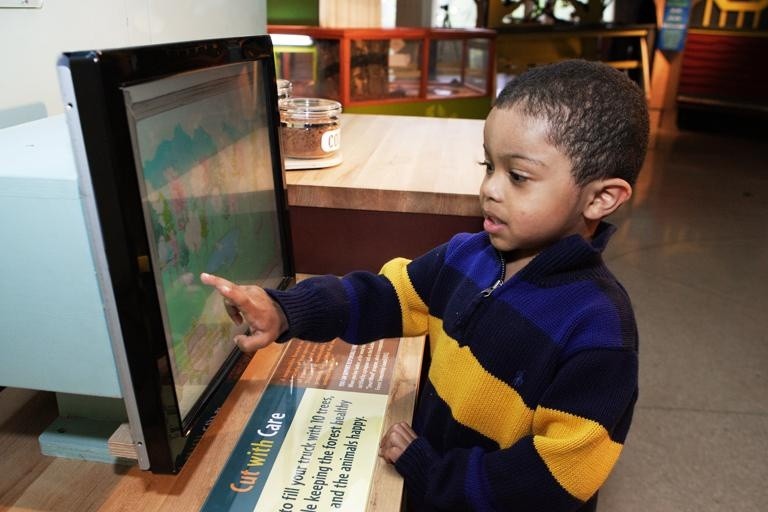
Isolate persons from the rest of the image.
[196,56,650,510]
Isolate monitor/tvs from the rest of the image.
[0,35,297,477]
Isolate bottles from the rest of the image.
[278,96,343,159]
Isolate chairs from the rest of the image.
[702,0,768,29]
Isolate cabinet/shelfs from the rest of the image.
[267,25,496,119]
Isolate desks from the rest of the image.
[95,274,427,512]
